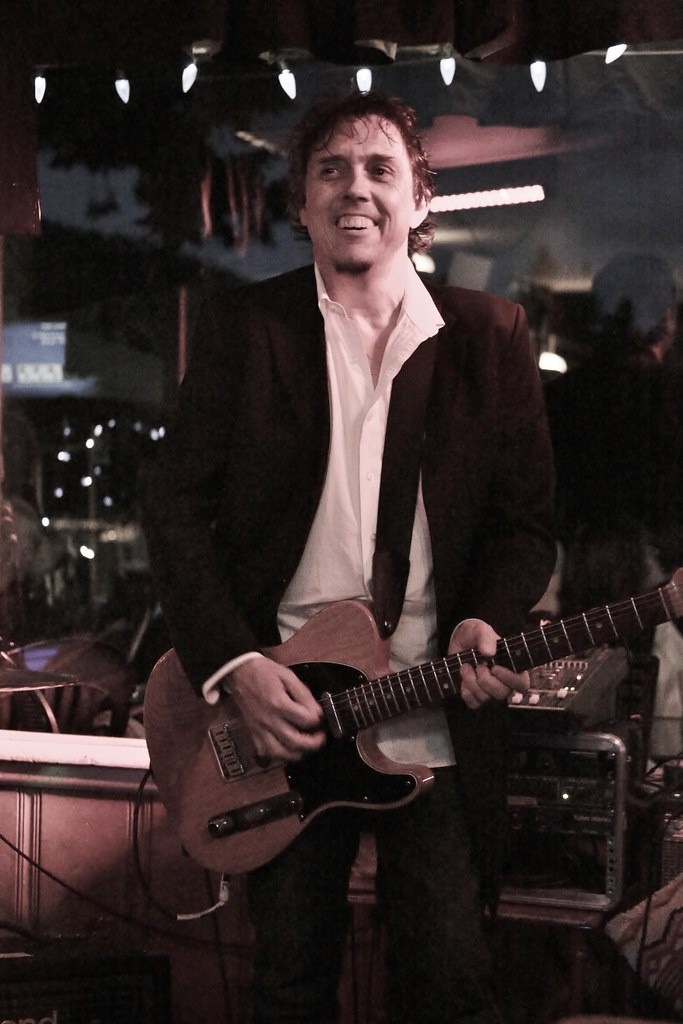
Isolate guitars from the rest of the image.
[141,566,682,874]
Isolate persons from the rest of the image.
[513,252,683,638]
[0,426,147,649]
[144,91,553,1024]
